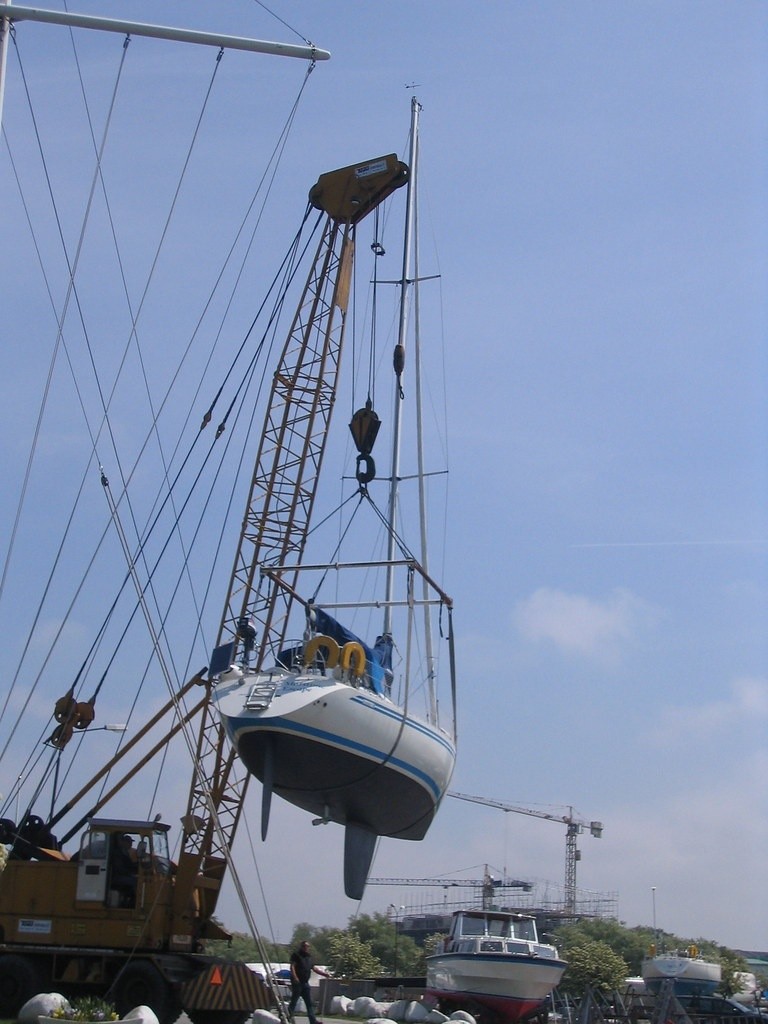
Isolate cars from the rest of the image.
[664,993,768,1024]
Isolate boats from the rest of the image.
[641,944,722,995]
[422,907,571,1023]
[207,636,461,901]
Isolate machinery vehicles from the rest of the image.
[1,143,412,1024]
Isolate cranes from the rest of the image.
[363,863,535,912]
[445,789,604,923]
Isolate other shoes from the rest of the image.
[310,1020,323,1024]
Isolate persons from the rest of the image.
[111,835,138,908]
[286,941,331,1024]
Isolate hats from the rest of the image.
[121,836,135,841]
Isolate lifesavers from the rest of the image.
[300,636,342,669]
[341,640,368,674]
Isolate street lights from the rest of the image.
[650,886,657,947]
[390,903,405,976]
[48,722,128,822]
